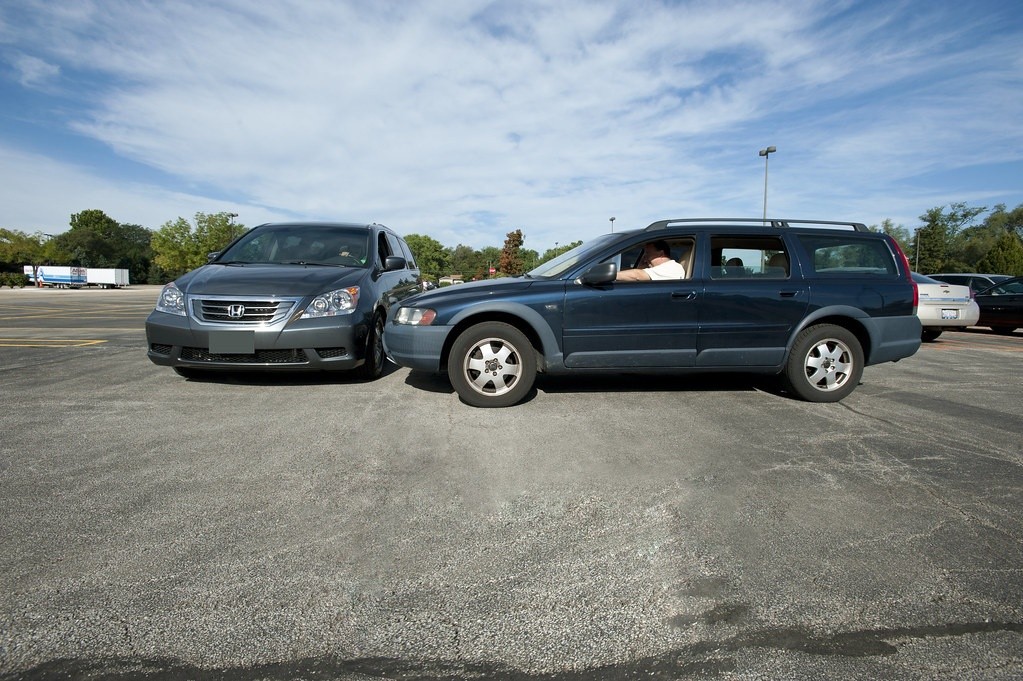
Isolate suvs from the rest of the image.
[384,217,922,409]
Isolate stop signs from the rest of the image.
[488,268,496,274]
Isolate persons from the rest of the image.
[338,245,351,258]
[614,239,686,281]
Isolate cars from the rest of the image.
[146,222,422,379]
[813,267,980,343]
[972,275,1023,333]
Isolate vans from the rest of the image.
[926,273,1023,295]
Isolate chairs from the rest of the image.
[716,257,750,280]
[757,253,789,279]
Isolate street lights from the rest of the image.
[555,242,558,257]
[609,217,616,234]
[759,146,777,226]
